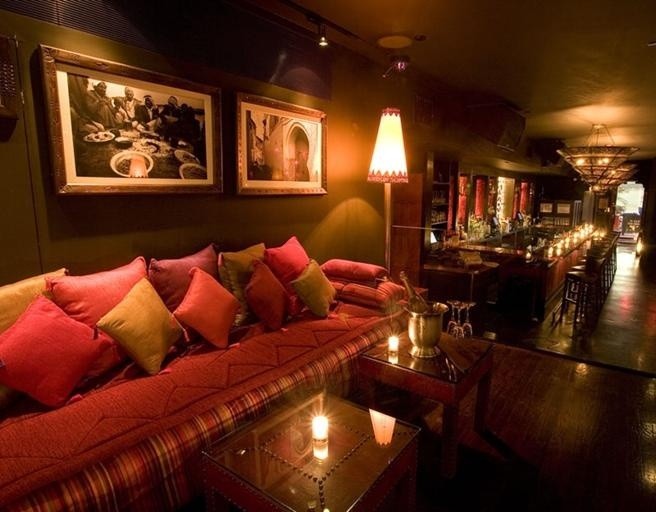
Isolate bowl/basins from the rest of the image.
[115,137,134,148]
[111,151,153,177]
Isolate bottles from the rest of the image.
[400,270,431,314]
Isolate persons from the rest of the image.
[110,97,128,127]
[87,79,115,130]
[170,105,201,154]
[136,94,160,126]
[158,96,178,133]
[115,89,140,118]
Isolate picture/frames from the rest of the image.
[38,44,224,196]
[232,91,328,197]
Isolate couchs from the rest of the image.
[1,288,428,512]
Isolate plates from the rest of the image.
[179,163,208,180]
[82,131,115,143]
[174,149,200,163]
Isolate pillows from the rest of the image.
[148,244,219,357]
[290,258,337,319]
[264,236,309,319]
[319,258,387,281]
[172,267,240,350]
[0,267,67,408]
[327,276,404,308]
[45,256,146,388]
[0,295,112,411]
[95,277,183,376]
[244,260,287,330]
[217,242,265,328]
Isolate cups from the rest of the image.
[461,300,478,339]
[452,302,465,342]
[446,299,458,336]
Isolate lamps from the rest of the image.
[388,352,399,365]
[367,108,408,275]
[313,440,328,464]
[317,23,328,46]
[368,408,396,451]
[387,335,400,352]
[555,124,639,189]
[609,169,639,192]
[580,163,636,196]
[311,411,328,440]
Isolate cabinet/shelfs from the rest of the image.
[423,246,524,320]
[502,229,594,323]
[424,151,529,278]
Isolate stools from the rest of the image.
[561,244,612,342]
